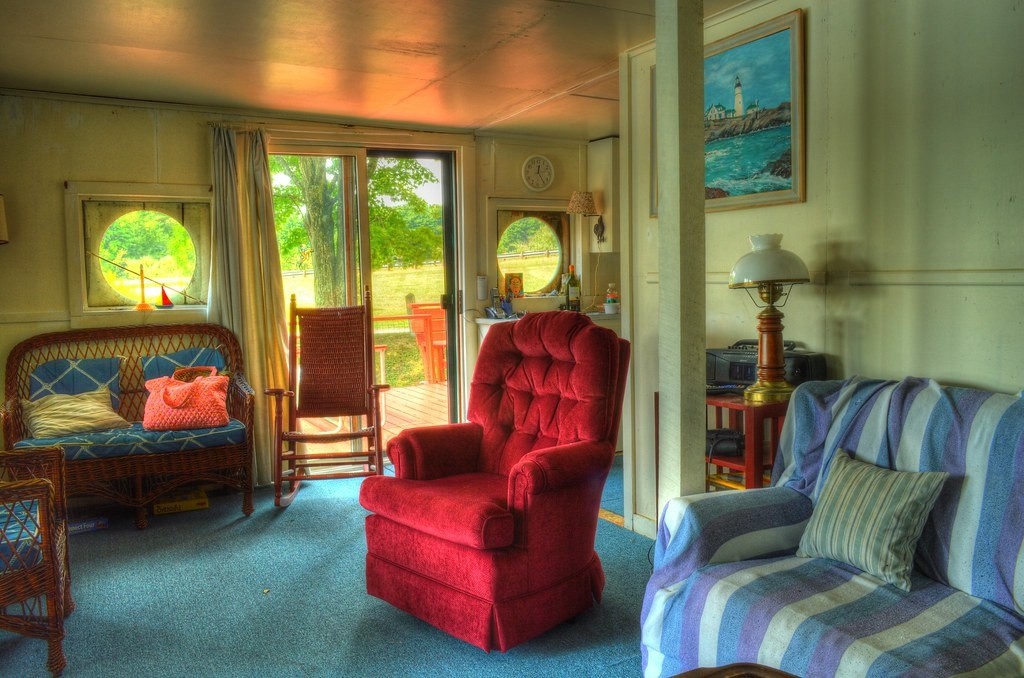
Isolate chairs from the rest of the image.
[296,344,388,433]
[262,284,391,508]
[0,445,75,678]
[358,310,633,654]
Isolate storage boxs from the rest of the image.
[706,472,771,493]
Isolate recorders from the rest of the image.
[706,339,828,394]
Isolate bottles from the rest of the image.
[606,282,618,303]
[567,265,581,311]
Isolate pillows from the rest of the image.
[30,357,120,410]
[22,384,134,440]
[796,446,950,592]
[141,347,225,395]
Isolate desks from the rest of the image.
[654,391,789,488]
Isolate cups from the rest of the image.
[603,303,619,314]
[502,302,512,316]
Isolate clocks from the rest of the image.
[522,155,555,192]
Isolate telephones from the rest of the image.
[484,286,507,319]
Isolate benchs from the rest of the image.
[409,302,447,385]
[0,323,257,530]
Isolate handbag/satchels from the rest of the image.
[142,366,230,431]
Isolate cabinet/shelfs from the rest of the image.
[476,313,621,351]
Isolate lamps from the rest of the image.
[728,233,811,402]
[565,190,605,244]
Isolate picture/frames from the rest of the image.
[648,7,808,220]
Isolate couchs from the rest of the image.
[639,375,1024,678]
